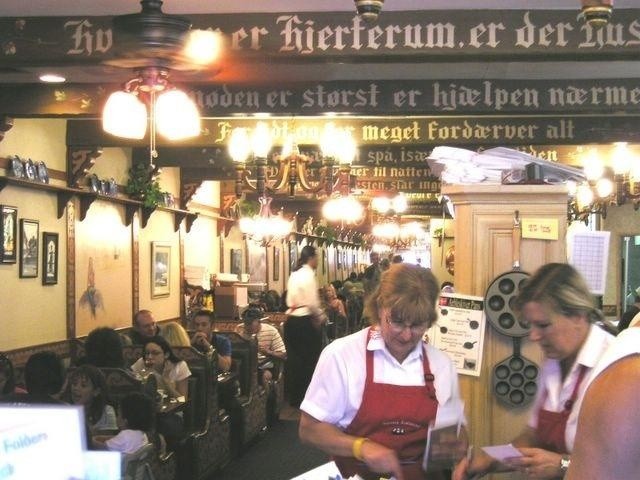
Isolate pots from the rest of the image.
[490,332,541,412]
[483,215,537,340]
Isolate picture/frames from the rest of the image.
[0,204,19,264]
[288,242,297,275]
[149,240,171,299]
[18,218,41,279]
[320,248,358,277]
[272,245,280,282]
[230,247,241,282]
[41,230,60,286]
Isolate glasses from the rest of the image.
[383,306,431,334]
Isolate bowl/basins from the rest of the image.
[217,280,240,286]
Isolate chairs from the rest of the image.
[248,289,281,312]
[1,391,69,406]
[324,293,363,347]
[66,311,286,480]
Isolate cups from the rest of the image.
[241,274,251,282]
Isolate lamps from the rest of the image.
[227,116,362,201]
[99,68,203,147]
[563,142,640,227]
[372,214,425,250]
[238,196,292,246]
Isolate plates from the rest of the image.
[11,154,48,183]
[89,173,118,199]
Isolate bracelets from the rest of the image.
[560,454,570,475]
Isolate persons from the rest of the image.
[617,286,640,331]
[563,327,639,480]
[298,264,470,480]
[449,262,621,480]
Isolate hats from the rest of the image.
[241,308,262,322]
